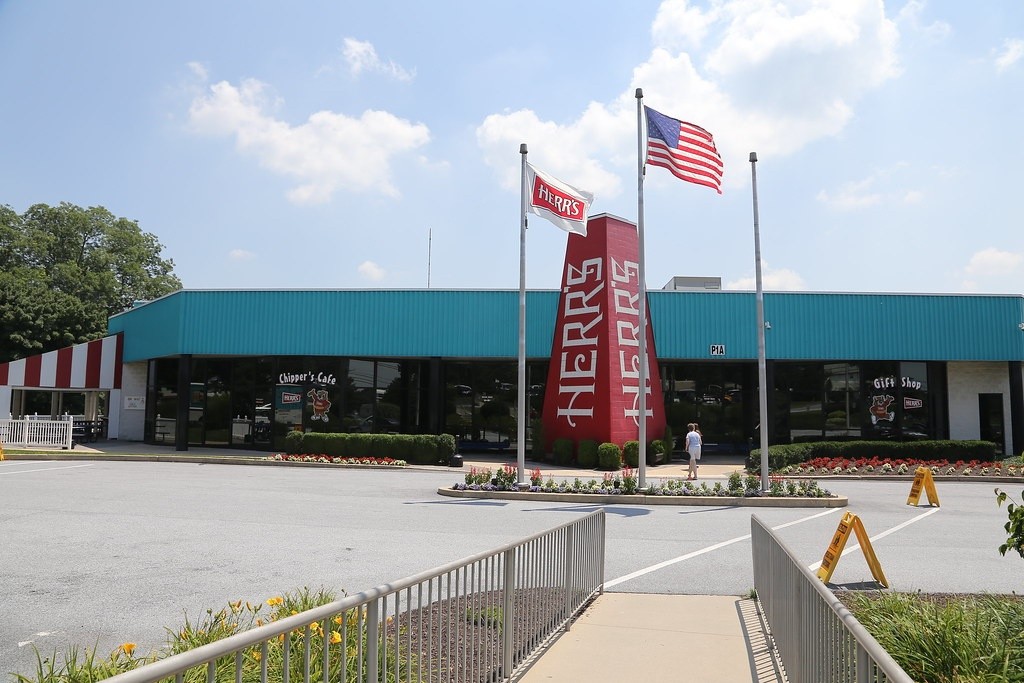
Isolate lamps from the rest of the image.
[200,377,227,400]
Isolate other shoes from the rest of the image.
[693,477,698,480]
[687,477,692,480]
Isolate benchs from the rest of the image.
[27,434,86,437]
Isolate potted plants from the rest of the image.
[647,440,664,462]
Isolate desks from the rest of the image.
[18,420,104,449]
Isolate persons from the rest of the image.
[685,424,702,481]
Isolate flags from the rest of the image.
[524,163,594,237]
[644,105,725,195]
